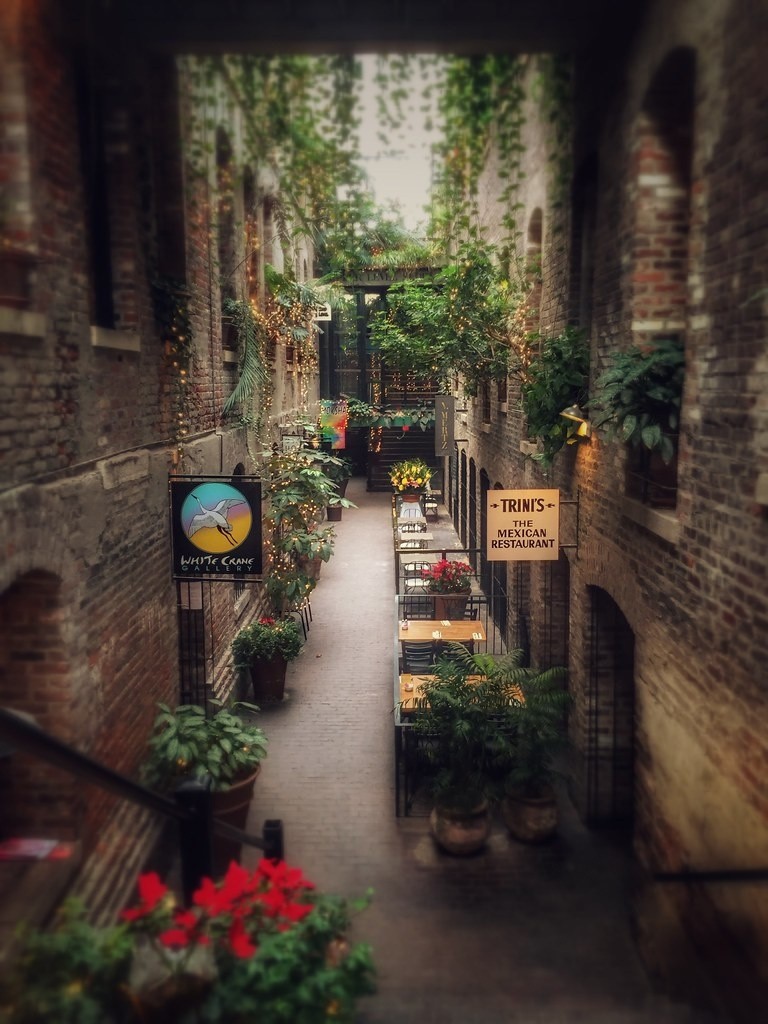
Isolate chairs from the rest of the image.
[391,490,519,818]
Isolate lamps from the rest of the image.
[560,398,585,422]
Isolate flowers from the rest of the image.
[419,559,476,594]
[0,855,382,1024]
[388,458,438,491]
[230,616,305,673]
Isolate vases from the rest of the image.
[402,493,420,503]
[249,654,288,704]
[427,585,472,620]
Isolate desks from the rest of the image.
[401,533,433,542]
[398,620,487,675]
[398,676,531,718]
[401,554,438,565]
[394,490,442,498]
[397,517,427,525]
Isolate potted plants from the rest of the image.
[325,444,354,498]
[262,569,316,613]
[276,524,338,581]
[147,694,269,878]
[389,673,518,857]
[433,641,572,843]
[326,486,343,522]
